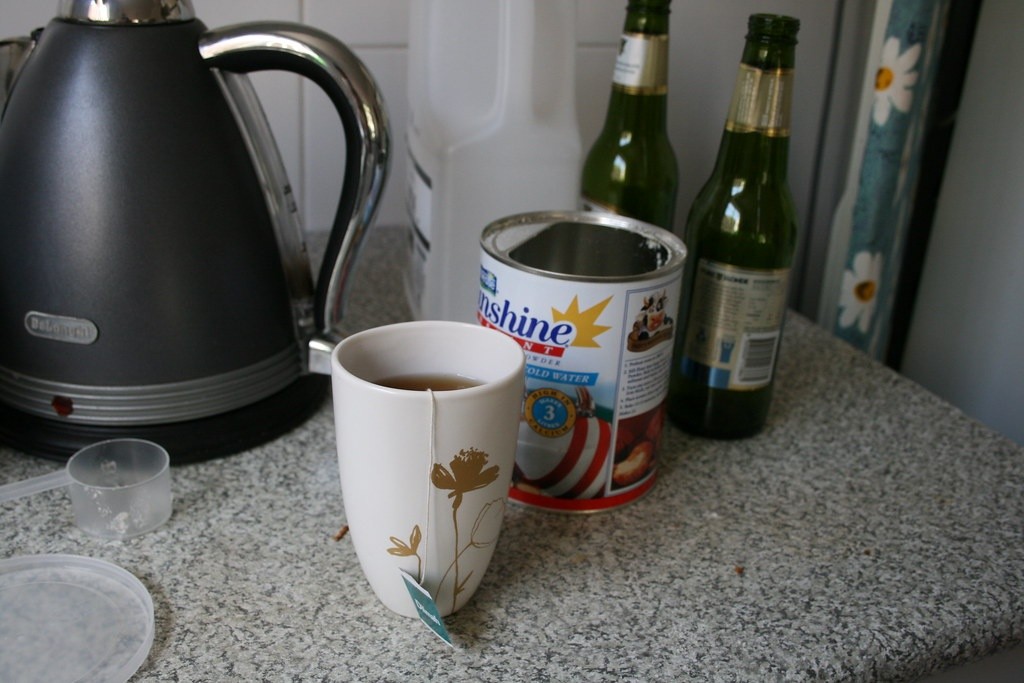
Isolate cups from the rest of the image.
[331,322,527,618]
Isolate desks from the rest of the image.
[0,224,1024,683]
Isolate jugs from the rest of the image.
[0,0,395,473]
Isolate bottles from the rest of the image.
[408,1,583,322]
[578,1,680,232]
[668,14,801,441]
